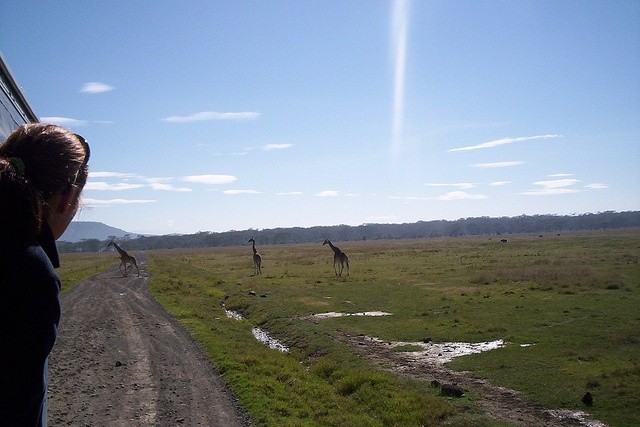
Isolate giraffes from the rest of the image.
[108,240,141,278]
[248,237,263,276]
[323,239,350,277]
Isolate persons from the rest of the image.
[1,123,90,427]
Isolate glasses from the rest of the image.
[72,132,90,183]
